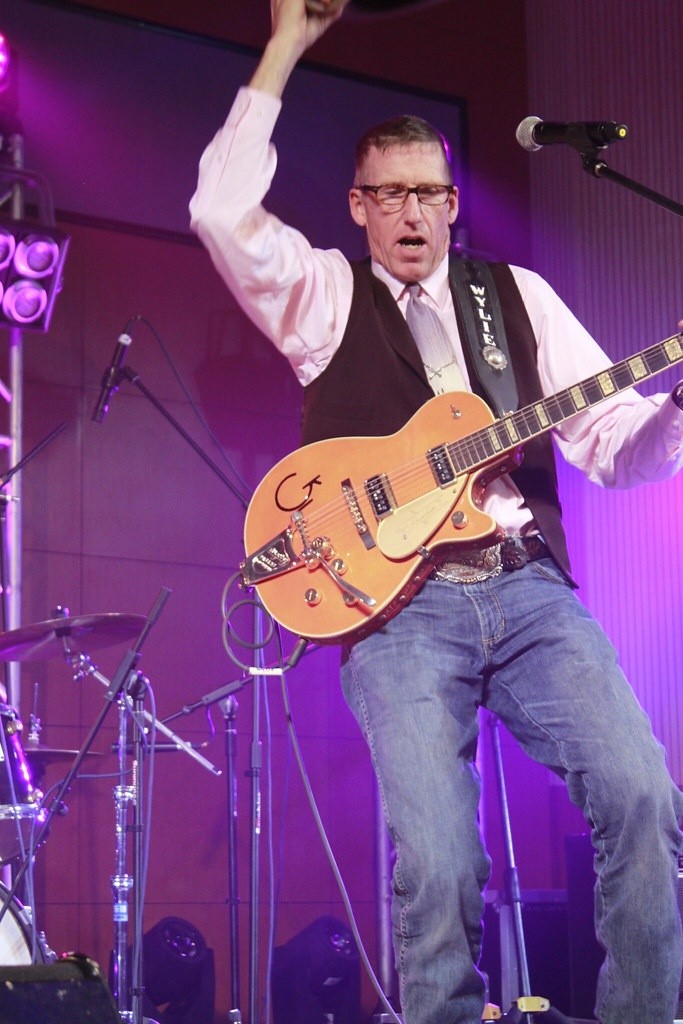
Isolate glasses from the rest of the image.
[355,182,453,207]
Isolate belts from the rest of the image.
[440,534,551,572]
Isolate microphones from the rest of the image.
[111,741,208,754]
[516,116,629,151]
[92,320,133,423]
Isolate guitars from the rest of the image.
[243,329,683,647]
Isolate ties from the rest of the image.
[405,280,471,398]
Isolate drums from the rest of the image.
[0,703,54,868]
[1,879,49,966]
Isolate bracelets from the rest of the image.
[675,385,683,411]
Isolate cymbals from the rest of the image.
[0,612,149,664]
[24,744,109,765]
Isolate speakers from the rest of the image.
[0,960,122,1024]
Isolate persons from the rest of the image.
[187,1,683,1024]
